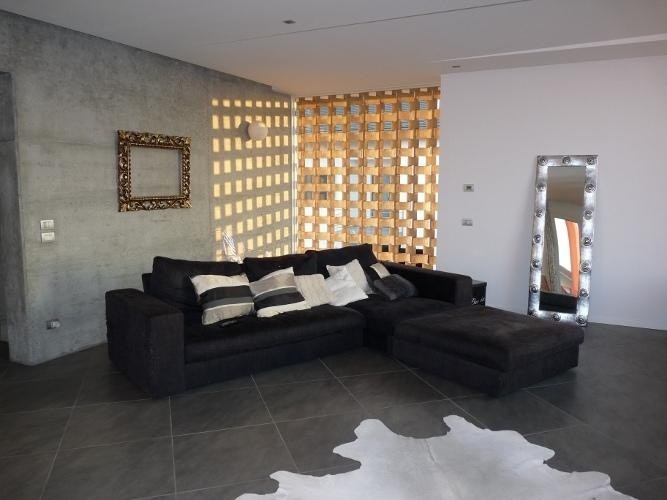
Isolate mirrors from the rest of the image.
[525,153,599,327]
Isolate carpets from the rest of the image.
[233,413,641,500]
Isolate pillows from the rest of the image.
[188,255,418,326]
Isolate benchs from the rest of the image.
[392,305,585,397]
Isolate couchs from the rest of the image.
[105,241,474,402]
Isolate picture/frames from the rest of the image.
[115,128,191,213]
